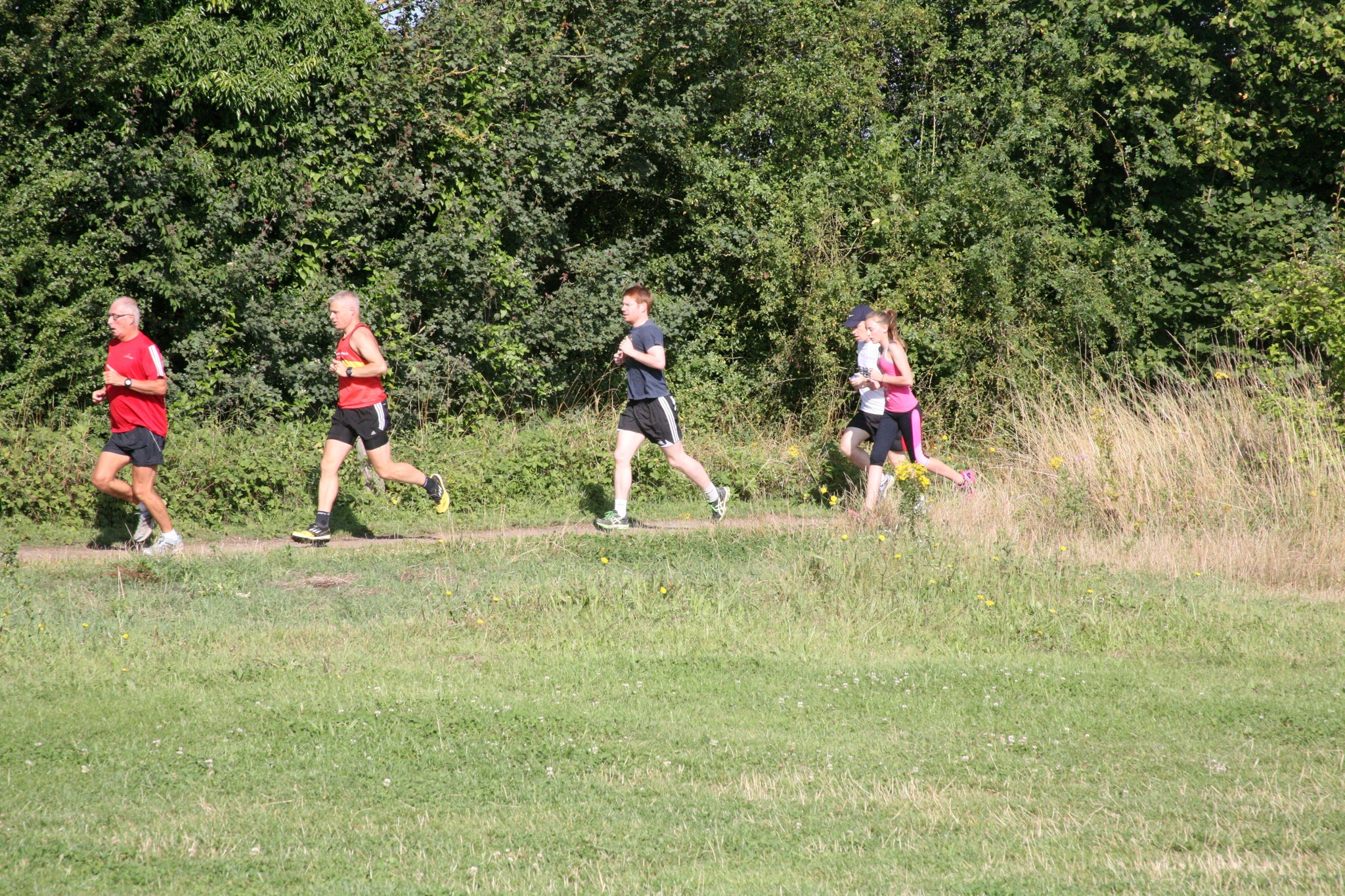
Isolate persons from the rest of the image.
[840,305,934,523]
[290,291,449,544]
[592,286,730,532]
[854,309,975,534]
[89,296,185,556]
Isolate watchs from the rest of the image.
[345,367,354,378]
[125,377,132,389]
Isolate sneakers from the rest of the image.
[957,469,976,497]
[708,486,731,524]
[142,534,184,555]
[595,509,629,530]
[132,509,155,542]
[878,474,894,499]
[290,522,330,543]
[429,474,450,513]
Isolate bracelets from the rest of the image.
[881,375,884,382]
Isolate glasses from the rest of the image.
[106,314,130,321]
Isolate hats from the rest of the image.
[842,305,874,327]
